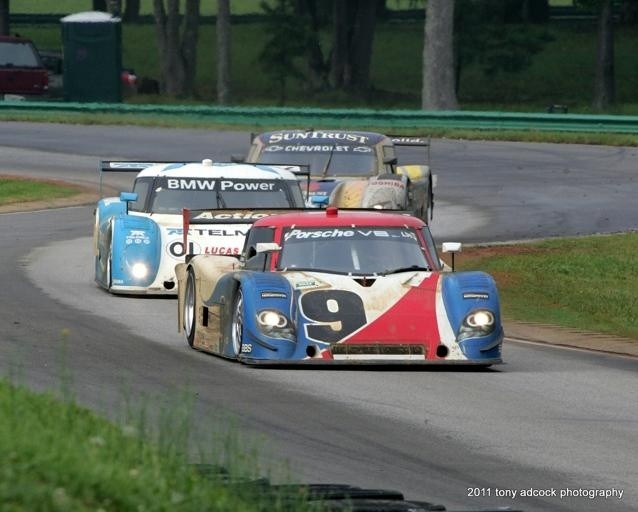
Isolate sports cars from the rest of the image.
[231,127,434,227]
[175,206,505,368]
[93,158,310,296]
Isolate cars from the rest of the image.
[0,32,50,98]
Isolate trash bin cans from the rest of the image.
[60,11,123,103]
